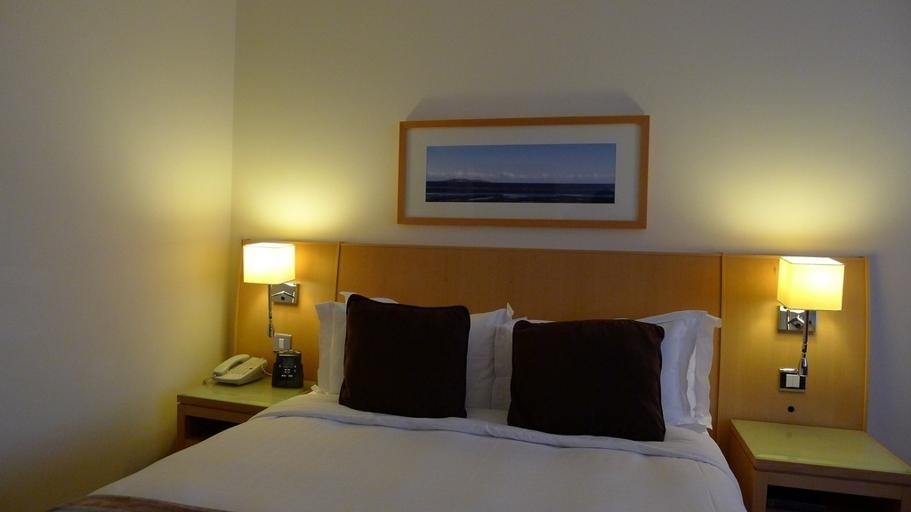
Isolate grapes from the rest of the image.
[177,376,318,451]
[727,418,911,512]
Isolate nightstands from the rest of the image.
[727,418,911,512]
[177,376,318,451]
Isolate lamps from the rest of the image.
[242,242,296,337]
[777,255,845,377]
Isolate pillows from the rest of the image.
[493,310,721,434]
[507,319,667,440]
[338,295,470,417]
[318,293,514,409]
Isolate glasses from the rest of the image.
[242,242,296,337]
[777,255,845,377]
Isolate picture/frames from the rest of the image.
[397,114,650,230]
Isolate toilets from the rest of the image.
[212,354,268,385]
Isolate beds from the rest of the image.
[43,241,749,512]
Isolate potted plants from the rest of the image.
[397,114,650,230]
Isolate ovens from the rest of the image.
[43,241,749,512]
